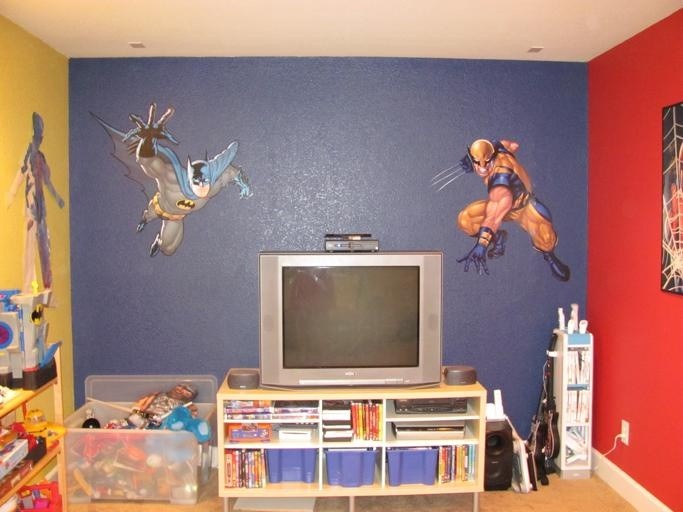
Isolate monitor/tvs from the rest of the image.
[258,250,443,390]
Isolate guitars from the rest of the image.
[542,334,559,460]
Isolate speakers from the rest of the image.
[445,366,476,385]
[228,370,259,389]
[484,419,513,491]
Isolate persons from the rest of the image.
[431,139,570,282]
[122,102,254,257]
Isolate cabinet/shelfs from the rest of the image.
[553,330,593,479]
[214,367,485,511]
[0,343,63,512]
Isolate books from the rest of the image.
[566,347,589,424]
[439,445,477,485]
[322,399,383,443]
[225,448,266,489]
[224,400,320,444]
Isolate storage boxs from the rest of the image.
[63,399,212,502]
